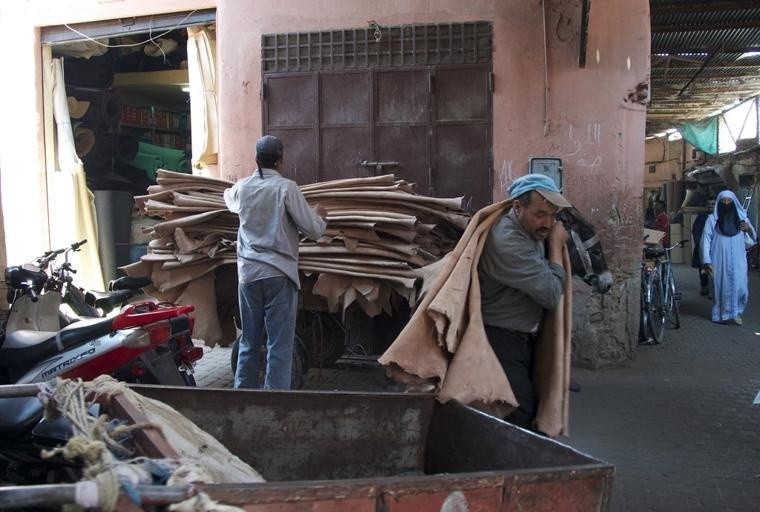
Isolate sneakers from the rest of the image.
[702,287,712,299]
[734,315,743,324]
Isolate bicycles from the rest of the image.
[642,233,666,344]
[745,225,759,273]
[643,238,692,345]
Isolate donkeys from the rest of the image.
[542,202,616,296]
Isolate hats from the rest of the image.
[507,174,573,208]
[256,136,283,158]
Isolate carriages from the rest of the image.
[214,196,615,394]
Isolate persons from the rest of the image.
[472,171,571,429]
[689,196,713,299]
[651,199,671,251]
[219,133,329,394]
[697,190,758,326]
